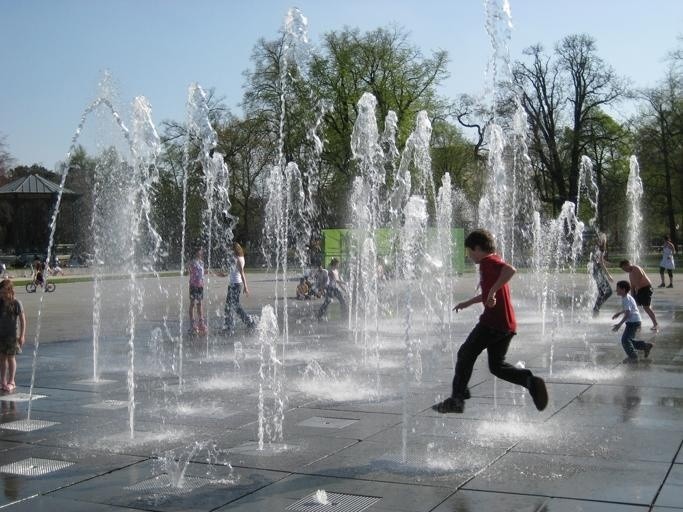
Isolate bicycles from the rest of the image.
[26,274,55,293]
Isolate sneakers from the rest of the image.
[623,357,638,364]
[644,343,653,357]
[528,376,549,411]
[431,397,465,414]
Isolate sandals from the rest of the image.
[3,383,16,391]
[0,384,5,390]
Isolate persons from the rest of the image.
[587,237,615,315]
[214,241,256,338]
[0,279,26,391]
[293,277,315,300]
[610,280,654,364]
[182,244,208,336]
[52,257,63,276]
[315,258,348,321]
[305,274,323,299]
[656,234,676,289]
[618,258,659,331]
[31,256,48,293]
[430,228,549,414]
[309,261,330,298]
